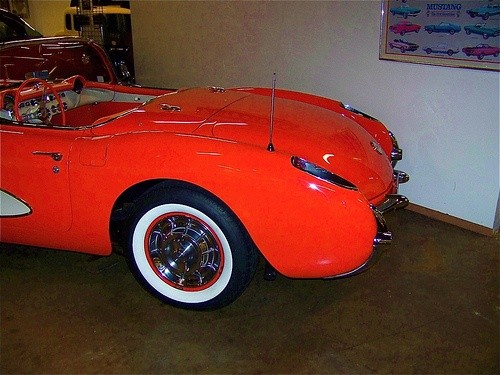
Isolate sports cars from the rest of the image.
[0,74,410,312]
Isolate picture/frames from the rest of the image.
[379,0,500,72]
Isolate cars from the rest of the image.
[0,8,119,90]
